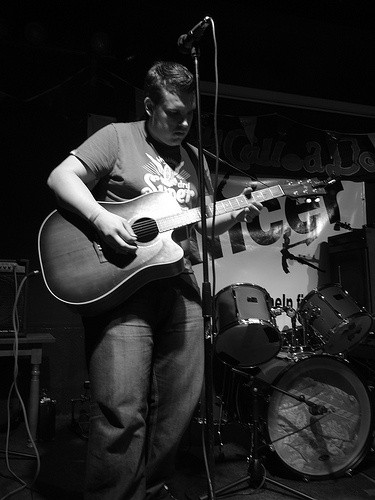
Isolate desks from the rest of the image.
[0,333,56,448]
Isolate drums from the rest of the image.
[295,283,372,354]
[279,325,322,352]
[210,282,282,368]
[236,352,375,480]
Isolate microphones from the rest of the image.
[308,407,335,416]
[177,16,211,47]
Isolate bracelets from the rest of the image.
[88,206,105,224]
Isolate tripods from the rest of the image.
[198,359,317,500]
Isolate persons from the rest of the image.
[46,61,262,499]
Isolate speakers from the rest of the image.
[0,259,29,338]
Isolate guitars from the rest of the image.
[37,177,328,317]
